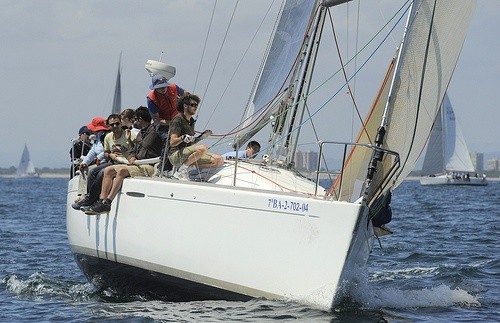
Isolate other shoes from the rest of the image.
[72,193,92,210]
[173,171,190,182]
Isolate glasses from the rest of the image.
[110,122,120,127]
[135,118,138,121]
[186,102,198,108]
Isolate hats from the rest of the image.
[86,116,110,131]
[148,75,172,90]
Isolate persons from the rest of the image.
[222,141,261,160]
[167,94,224,180]
[147,75,187,126]
[70,106,163,215]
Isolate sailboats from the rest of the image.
[14,143,41,179]
[418,91,491,188]
[64,0,478,314]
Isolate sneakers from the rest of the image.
[80,201,111,215]
